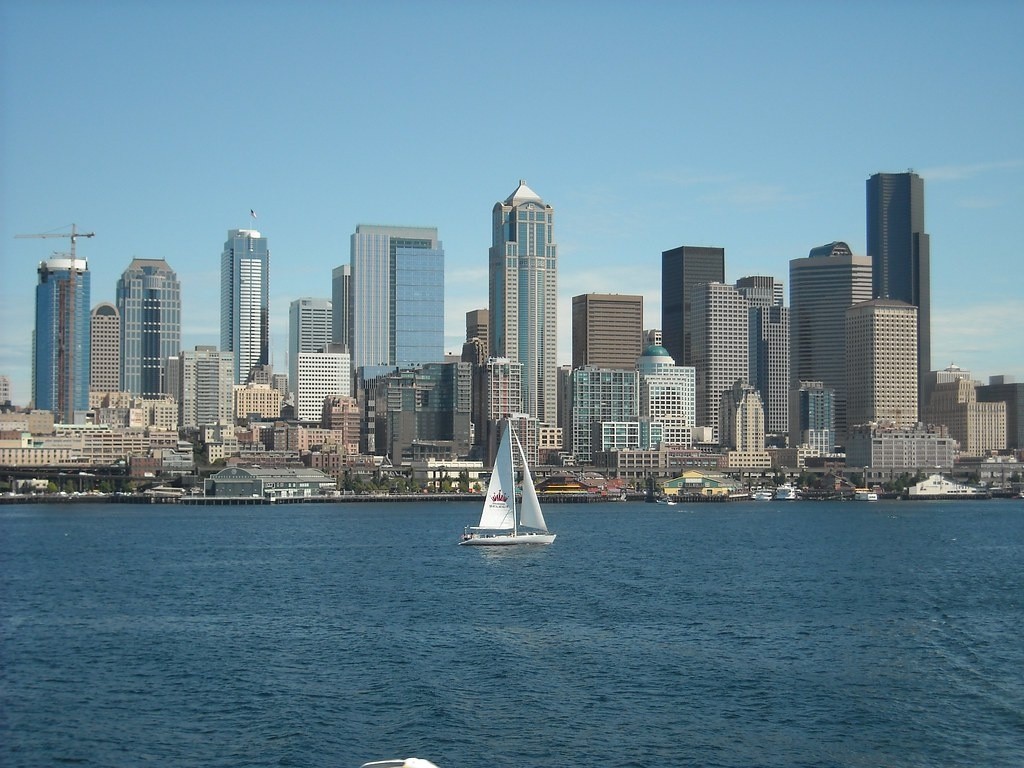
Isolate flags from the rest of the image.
[251,210,256,218]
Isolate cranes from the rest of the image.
[13,223,97,423]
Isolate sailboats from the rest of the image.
[458,420,557,545]
